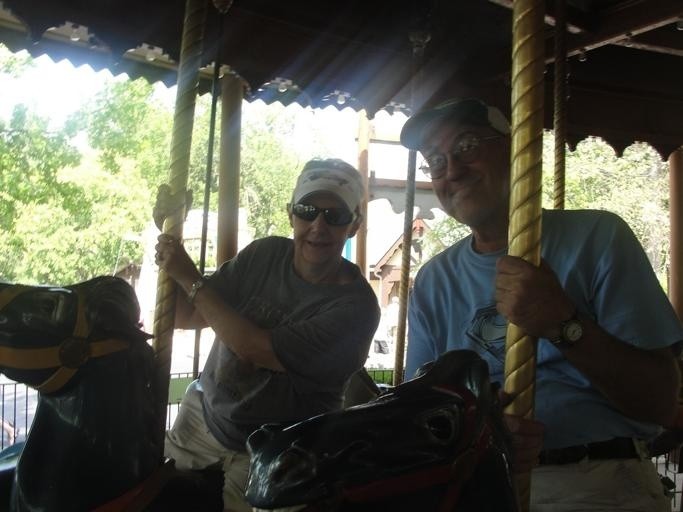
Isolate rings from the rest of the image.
[156,252,161,261]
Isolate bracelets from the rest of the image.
[186,275,209,307]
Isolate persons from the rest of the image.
[1,416,19,447]
[399,95,683,512]
[151,154,381,511]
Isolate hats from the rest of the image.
[291,167,368,214]
[402,97,511,152]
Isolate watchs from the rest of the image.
[549,306,587,348]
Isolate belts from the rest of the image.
[535,437,636,465]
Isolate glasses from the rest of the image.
[291,203,358,226]
[419,129,506,178]
